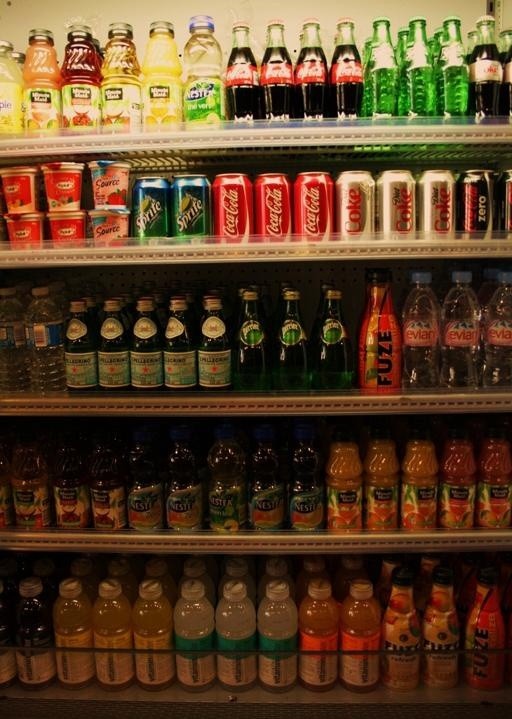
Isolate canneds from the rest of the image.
[131,170,511,246]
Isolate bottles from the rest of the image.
[0,15,512,139]
[1,269,512,693]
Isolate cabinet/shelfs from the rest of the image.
[0,116,512,719]
